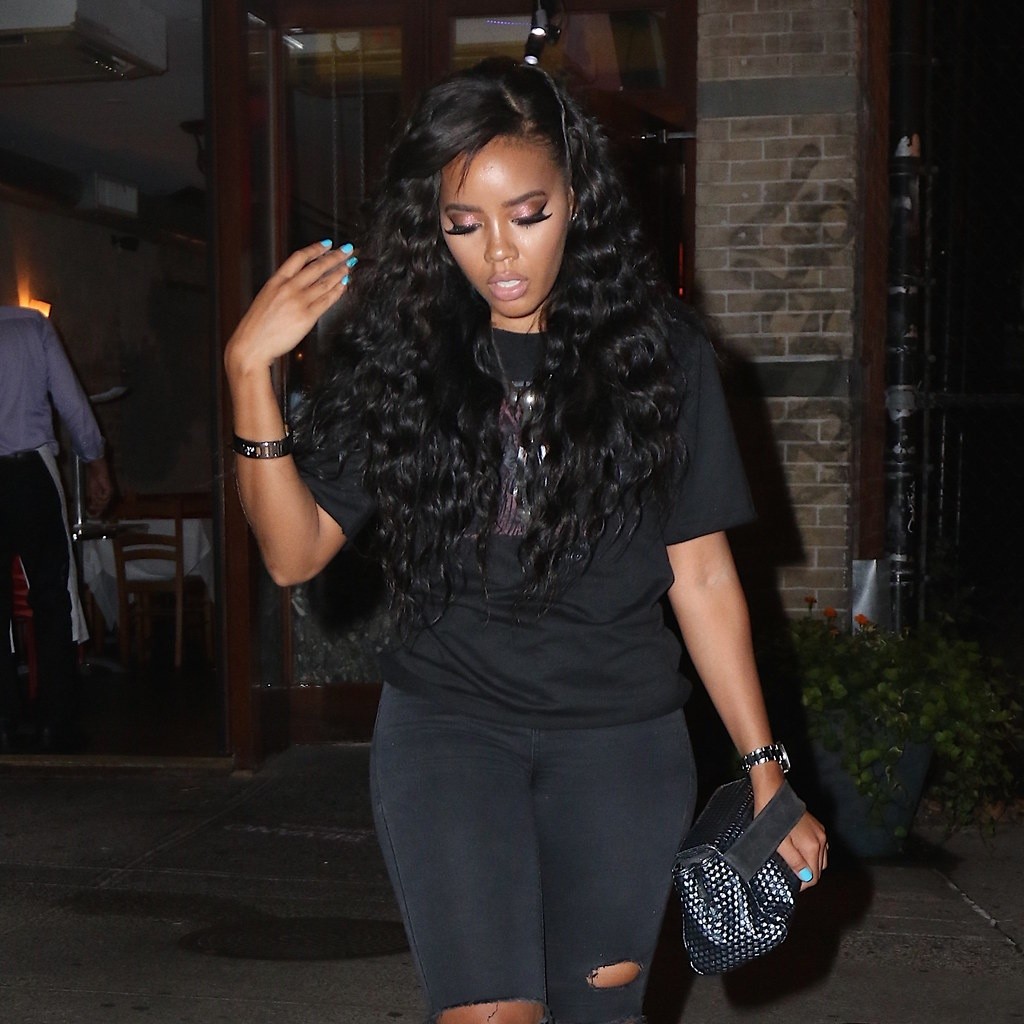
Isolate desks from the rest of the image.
[5,517,214,664]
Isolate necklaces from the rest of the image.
[488,327,512,394]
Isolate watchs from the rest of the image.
[740,741,791,776]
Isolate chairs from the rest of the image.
[108,497,214,672]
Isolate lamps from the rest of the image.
[523,0,567,65]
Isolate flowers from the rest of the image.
[753,595,1023,849]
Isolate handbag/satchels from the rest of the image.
[670,779,806,975]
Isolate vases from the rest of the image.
[790,728,935,864]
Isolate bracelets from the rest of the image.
[230,422,295,460]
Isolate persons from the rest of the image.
[225,58,829,1024]
[0,306,112,756]
